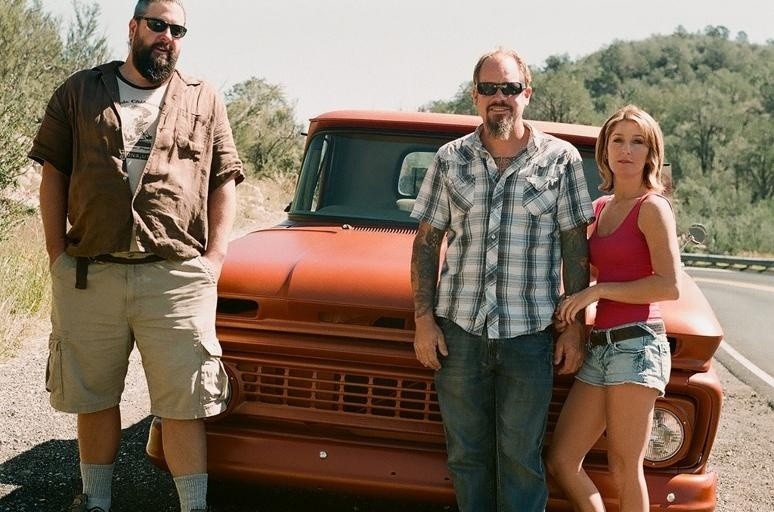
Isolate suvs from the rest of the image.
[143,110,724,511]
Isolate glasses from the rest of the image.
[475,81,527,96]
[136,15,187,39]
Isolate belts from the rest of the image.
[591,322,665,346]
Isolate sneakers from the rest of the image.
[67,493,113,512]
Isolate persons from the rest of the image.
[406,44,589,512]
[553,105,684,512]
[24,1,246,512]
